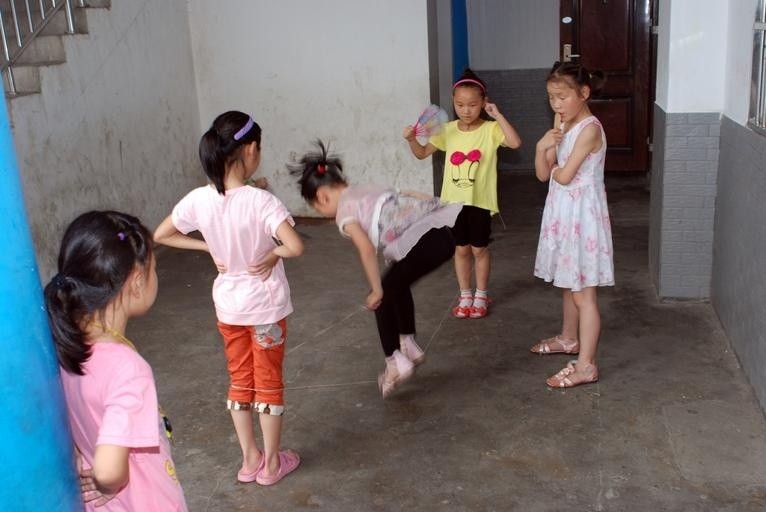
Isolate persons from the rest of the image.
[529,59,617,390]
[41,205,188,512]
[286,138,458,401]
[401,67,523,321]
[150,110,305,484]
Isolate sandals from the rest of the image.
[401,336,425,366]
[546,361,599,388]
[453,296,474,317]
[531,335,579,356]
[470,294,493,318]
[381,349,414,400]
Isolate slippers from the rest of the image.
[237,448,300,485]
